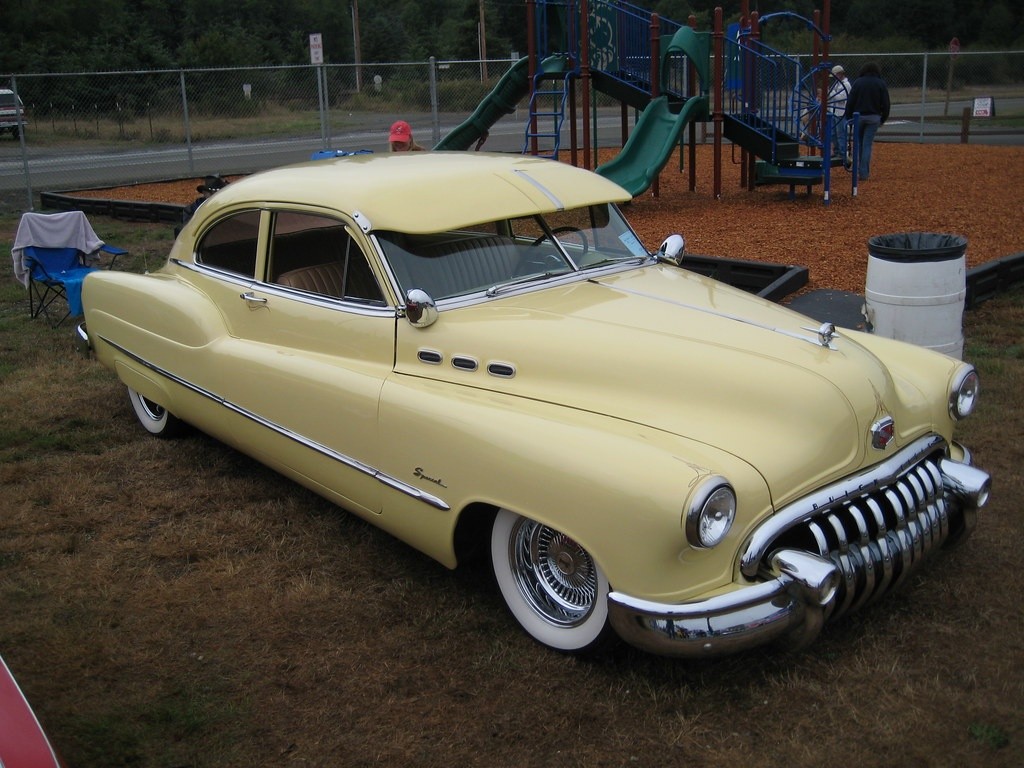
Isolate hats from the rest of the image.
[389,120,411,142]
[829,65,843,77]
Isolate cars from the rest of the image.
[77,151,992,655]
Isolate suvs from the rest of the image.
[0,88,27,138]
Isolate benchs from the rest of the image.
[280,236,524,307]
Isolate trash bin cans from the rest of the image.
[866,232,967,362]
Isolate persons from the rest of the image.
[389,120,426,152]
[183,173,229,233]
[802,61,890,181]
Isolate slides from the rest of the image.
[593,95,707,199]
[431,54,569,151]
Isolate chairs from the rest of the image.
[10,210,128,329]
